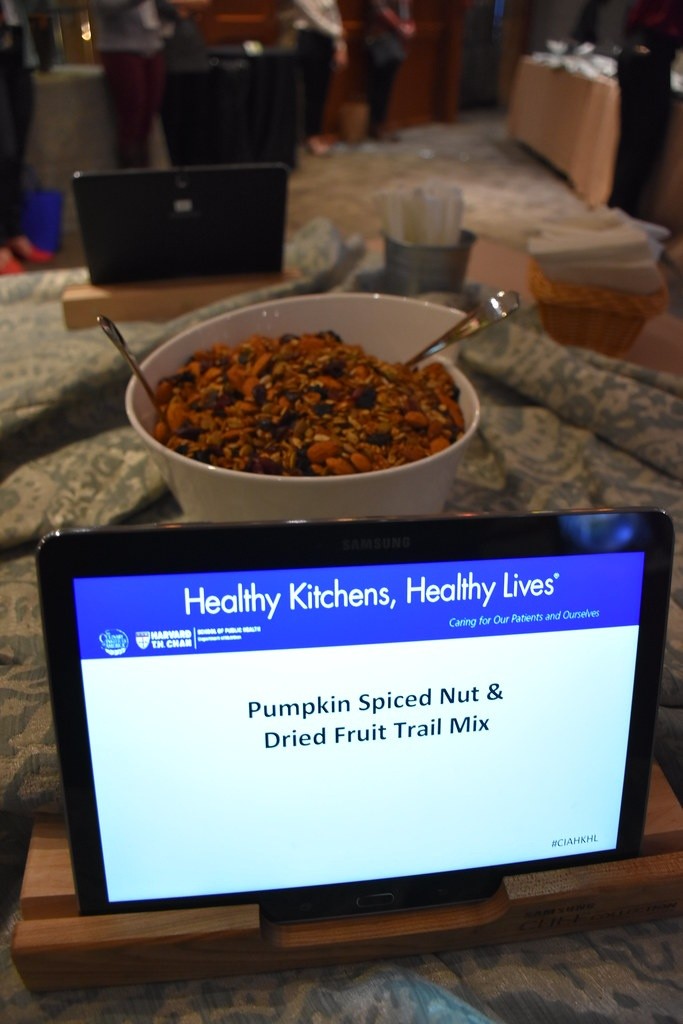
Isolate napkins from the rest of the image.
[527,207,669,294]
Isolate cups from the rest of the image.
[376,228,479,298]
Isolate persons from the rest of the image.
[94,0,221,172]
[363,0,416,142]
[0,0,53,275]
[27,0,57,76]
[606,0,683,211]
[276,0,348,154]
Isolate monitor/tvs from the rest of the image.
[32,503,675,929]
[69,159,288,286]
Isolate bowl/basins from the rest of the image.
[121,290,476,520]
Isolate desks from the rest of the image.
[1,267,683,1024]
[27,65,115,226]
[507,55,619,211]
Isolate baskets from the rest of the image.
[531,257,669,355]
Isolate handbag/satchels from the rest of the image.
[20,167,62,252]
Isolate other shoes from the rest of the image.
[0,247,26,274]
[12,234,53,264]
[307,134,332,158]
